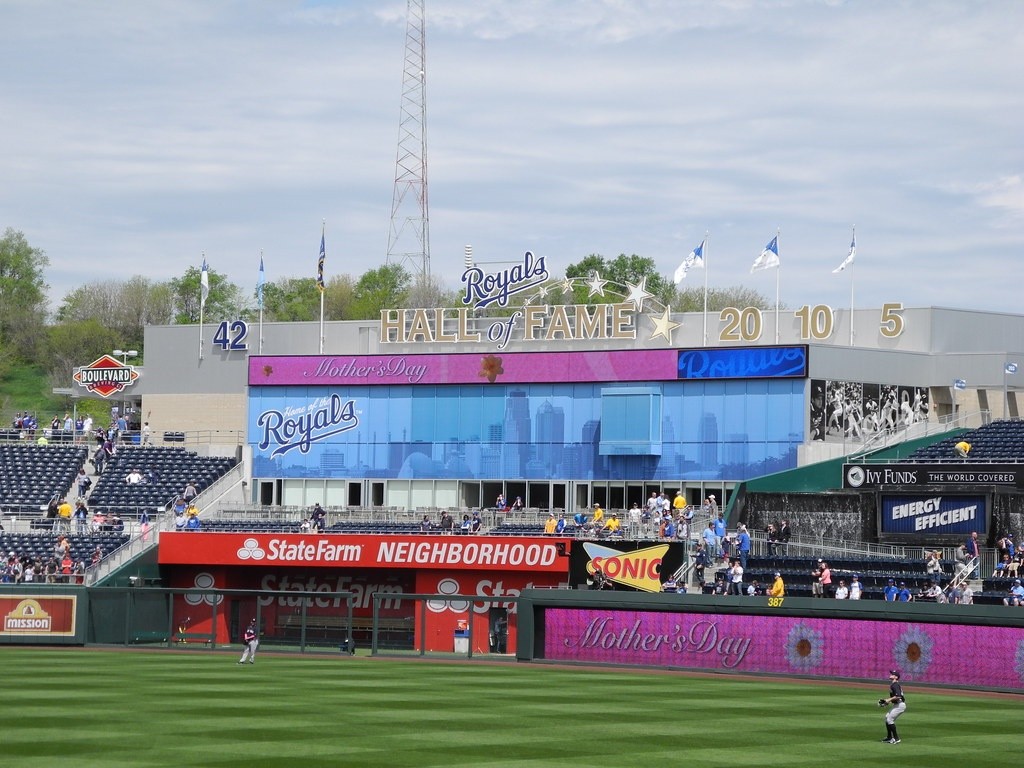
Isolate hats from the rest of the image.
[516,496,521,499]
[888,579,894,583]
[774,572,781,577]
[890,670,900,680]
[473,512,478,515]
[1015,579,1022,584]
[853,574,858,577]
[179,503,194,515]
[550,495,716,518]
[899,582,905,586]
[251,617,257,622]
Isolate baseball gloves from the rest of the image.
[242,640,249,645]
[878,698,890,707]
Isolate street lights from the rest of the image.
[112,350,137,420]
[70,396,81,445]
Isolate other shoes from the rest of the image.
[881,736,893,742]
[893,739,901,745]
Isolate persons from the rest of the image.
[237,619,259,664]
[513,496,525,512]
[419,515,432,535]
[461,512,482,535]
[496,494,507,511]
[300,503,328,533]
[494,617,507,653]
[811,379,933,443]
[878,669,907,745]
[812,531,1024,606]
[544,514,567,537]
[178,620,186,643]
[0,407,201,583]
[573,491,791,596]
[439,511,455,536]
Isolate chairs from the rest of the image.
[0,443,629,585]
[718,552,1024,606]
[898,419,1024,465]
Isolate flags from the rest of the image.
[201,255,209,307]
[259,254,265,310]
[674,239,705,285]
[832,229,857,274]
[751,235,781,274]
[316,226,327,293]
